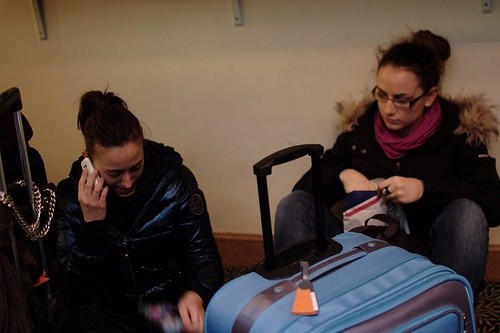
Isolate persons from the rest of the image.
[50,90,225,333]
[274,30,500,298]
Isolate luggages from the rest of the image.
[0,86,69,333]
[205,144,477,333]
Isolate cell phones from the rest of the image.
[81,157,104,191]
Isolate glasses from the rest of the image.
[372,86,424,108]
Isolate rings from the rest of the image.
[385,188,391,195]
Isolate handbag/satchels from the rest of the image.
[349,214,417,253]
[0,182,67,241]
